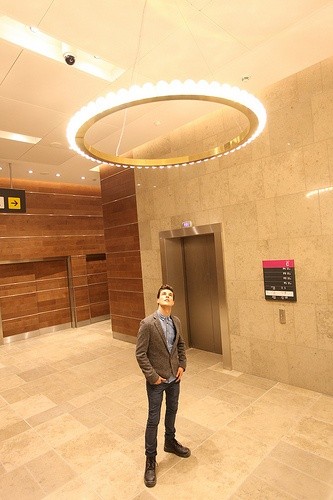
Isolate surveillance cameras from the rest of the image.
[63,53,75,66]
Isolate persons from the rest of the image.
[135,284,191,487]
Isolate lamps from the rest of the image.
[66,0,267,169]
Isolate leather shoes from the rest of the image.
[163,437,190,458]
[144,452,158,487]
